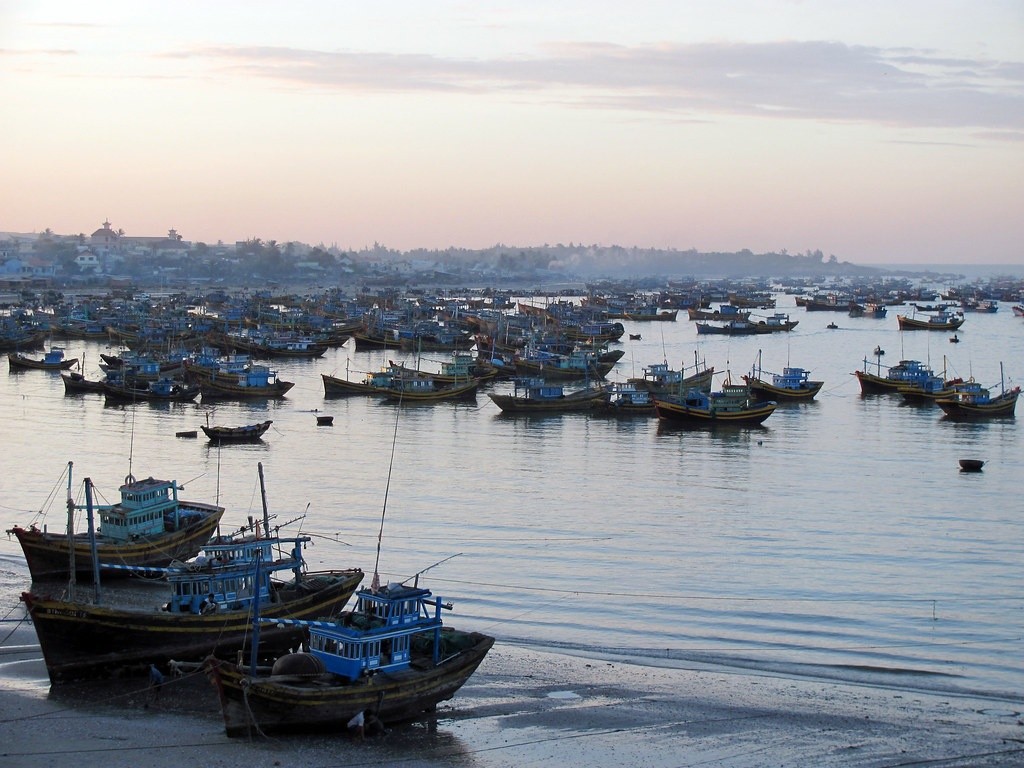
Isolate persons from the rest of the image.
[30,525,40,532]
[381,647,389,664]
[199,594,221,616]
[292,542,305,582]
[146,665,163,703]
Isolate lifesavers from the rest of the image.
[969,377,975,383]
[124,475,137,488]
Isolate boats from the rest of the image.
[3,266,1024,743]
